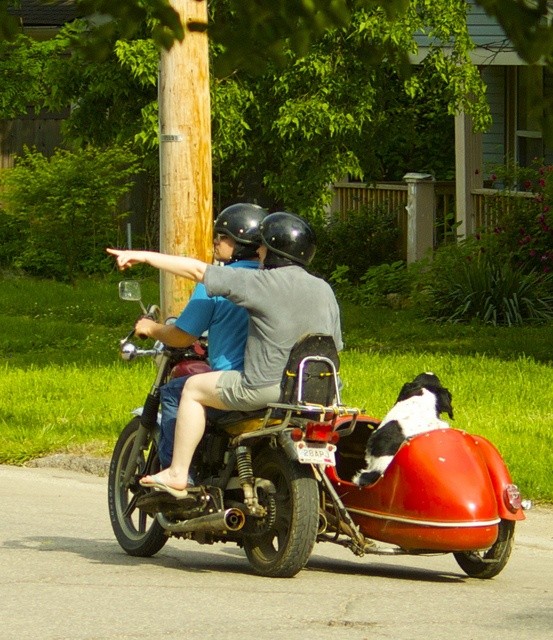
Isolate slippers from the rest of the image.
[139,475,188,499]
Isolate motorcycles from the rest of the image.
[106,278,366,579]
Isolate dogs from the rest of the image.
[352,371,456,489]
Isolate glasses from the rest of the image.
[216,233,227,241]
[255,239,265,245]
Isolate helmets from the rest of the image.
[259,212,318,268]
[213,203,269,259]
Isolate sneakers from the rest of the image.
[187,474,194,487]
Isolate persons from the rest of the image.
[135,203,276,494]
[104,211,343,501]
